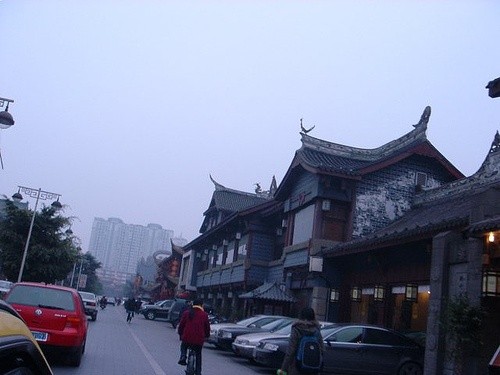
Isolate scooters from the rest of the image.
[101,302,106,310]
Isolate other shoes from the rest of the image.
[178,360,186,364]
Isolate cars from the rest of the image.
[0,280,13,296]
[0,299,55,375]
[167,297,192,329]
[204,314,337,369]
[78,291,116,321]
[252,321,425,375]
[3,282,90,368]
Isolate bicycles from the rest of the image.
[183,344,202,375]
[127,311,134,324]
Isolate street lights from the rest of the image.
[11,185,63,282]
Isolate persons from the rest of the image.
[100,296,107,307]
[149,299,154,305]
[125,298,136,321]
[282,307,326,375]
[178,298,210,375]
[135,298,142,315]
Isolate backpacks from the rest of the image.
[293,324,322,370]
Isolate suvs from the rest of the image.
[140,299,175,320]
